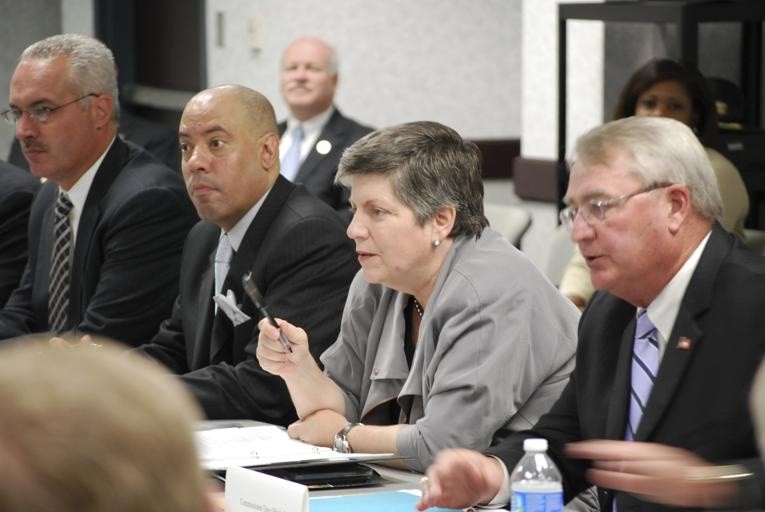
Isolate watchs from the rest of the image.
[332,422,364,454]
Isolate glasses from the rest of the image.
[559,181,673,233]
[0,93,99,124]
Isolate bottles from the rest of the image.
[508,438,568,511]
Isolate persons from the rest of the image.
[0,326,225,512]
[276,36,383,228]
[557,58,752,310]
[415,114,765,511]
[255,120,601,512]
[0,33,202,360]
[126,83,361,428]
[0,159,44,335]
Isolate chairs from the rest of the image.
[520,220,574,290]
[484,202,532,250]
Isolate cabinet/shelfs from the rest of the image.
[547,3,764,230]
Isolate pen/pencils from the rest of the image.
[241,273,293,352]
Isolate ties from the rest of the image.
[279,124,303,182]
[214,234,234,316]
[624,309,661,440]
[47,191,74,334]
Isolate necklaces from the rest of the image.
[413,291,425,318]
